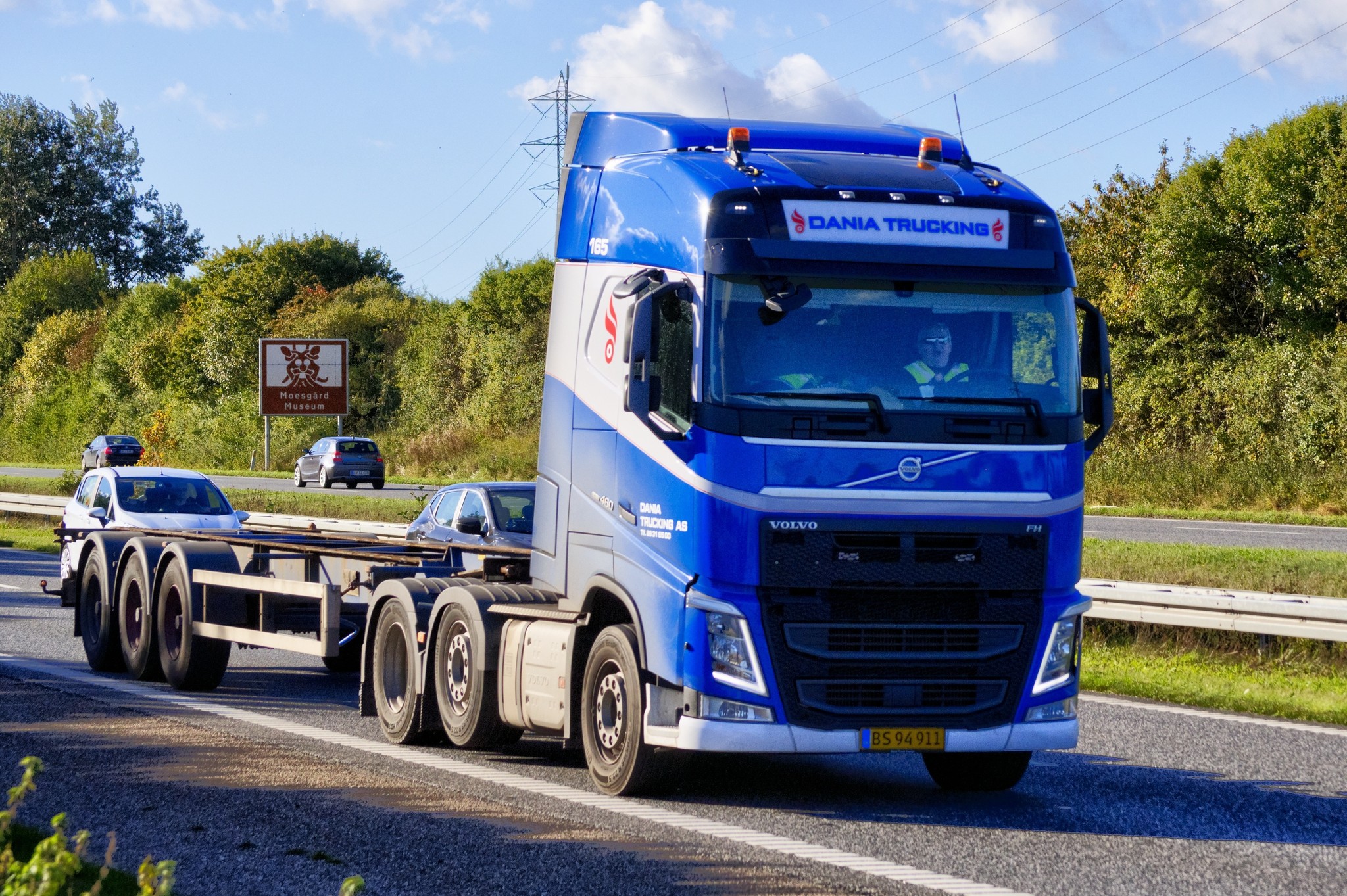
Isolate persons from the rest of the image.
[901,323,979,402]
[163,482,210,512]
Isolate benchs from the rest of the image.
[339,444,370,451]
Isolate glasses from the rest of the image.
[919,336,949,347]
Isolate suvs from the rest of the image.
[294,437,385,489]
[58,467,251,586]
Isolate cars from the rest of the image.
[81,436,145,472]
[405,482,536,573]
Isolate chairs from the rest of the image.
[144,488,170,509]
[118,482,141,512]
[489,496,511,529]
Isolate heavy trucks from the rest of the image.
[38,109,1115,800]
